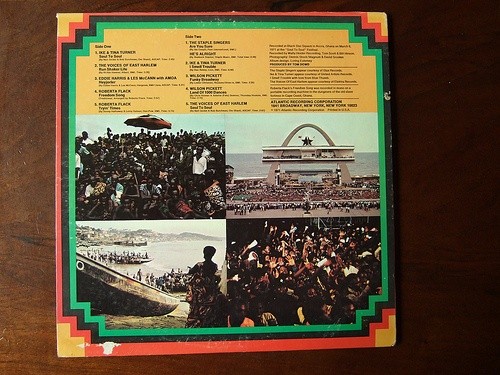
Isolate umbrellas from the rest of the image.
[125,114,172,134]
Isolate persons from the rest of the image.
[75,125,383,331]
